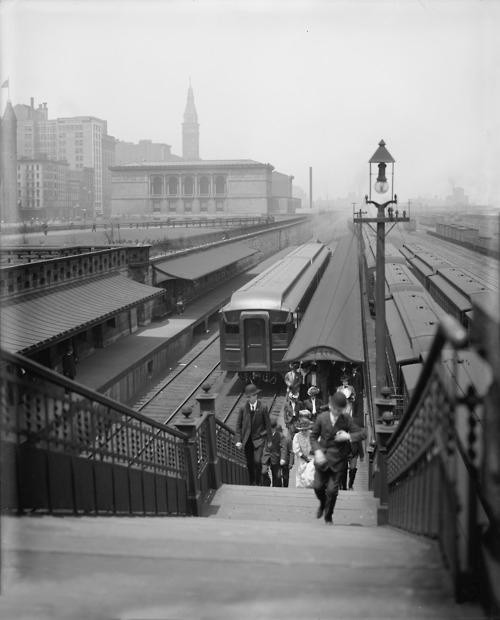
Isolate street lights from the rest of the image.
[353,138,411,399]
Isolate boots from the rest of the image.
[341,478,347,490]
[349,468,358,489]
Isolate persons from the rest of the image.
[291,417,316,488]
[262,417,288,486]
[283,417,302,471]
[276,425,295,486]
[174,291,185,317]
[61,344,78,396]
[309,390,368,525]
[234,383,271,486]
[283,358,365,432]
[339,413,365,491]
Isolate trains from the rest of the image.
[216,240,333,392]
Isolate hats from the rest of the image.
[245,384,262,396]
[289,391,299,400]
[307,387,320,396]
[340,375,350,381]
[295,419,314,430]
[328,392,348,410]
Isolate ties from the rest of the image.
[251,407,256,414]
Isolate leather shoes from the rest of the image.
[317,501,326,518]
[324,516,332,523]
